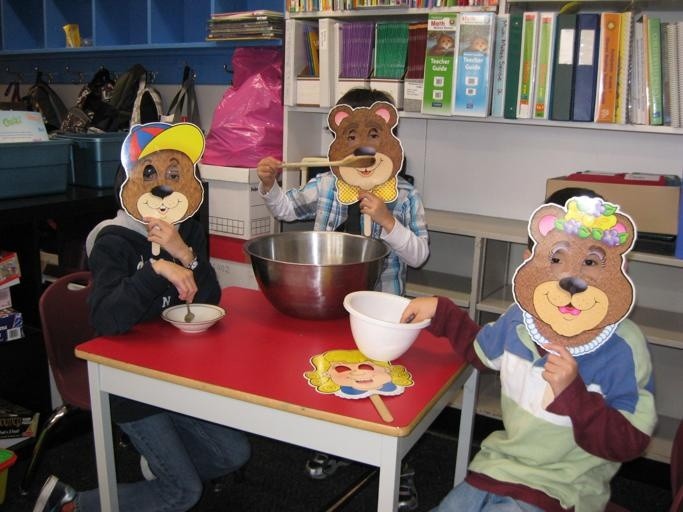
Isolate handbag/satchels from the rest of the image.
[27,72,69,132]
[110,64,147,111]
[60,86,95,134]
[127,77,163,129]
[82,95,120,133]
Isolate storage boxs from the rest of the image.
[193,159,283,241]
[50,130,140,192]
[202,230,267,294]
[0,137,78,198]
[543,169,681,254]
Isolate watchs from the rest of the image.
[183,251,200,274]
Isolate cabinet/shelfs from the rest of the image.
[0,1,286,86]
[404,200,682,480]
[277,0,683,208]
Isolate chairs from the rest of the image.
[600,420,683,511]
[18,270,163,498]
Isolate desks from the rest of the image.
[68,278,492,512]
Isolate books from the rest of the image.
[205,10,286,44]
[491,3,681,127]
[286,0,498,15]
[296,12,498,117]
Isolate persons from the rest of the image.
[33,121,256,510]
[257,89,432,509]
[400,188,660,511]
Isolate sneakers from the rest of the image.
[140,454,158,482]
[399,461,419,512]
[33,475,76,512]
[304,451,352,481]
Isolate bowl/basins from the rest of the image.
[241,230,394,321]
[159,303,226,333]
[342,289,432,362]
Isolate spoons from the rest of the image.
[182,300,196,323]
